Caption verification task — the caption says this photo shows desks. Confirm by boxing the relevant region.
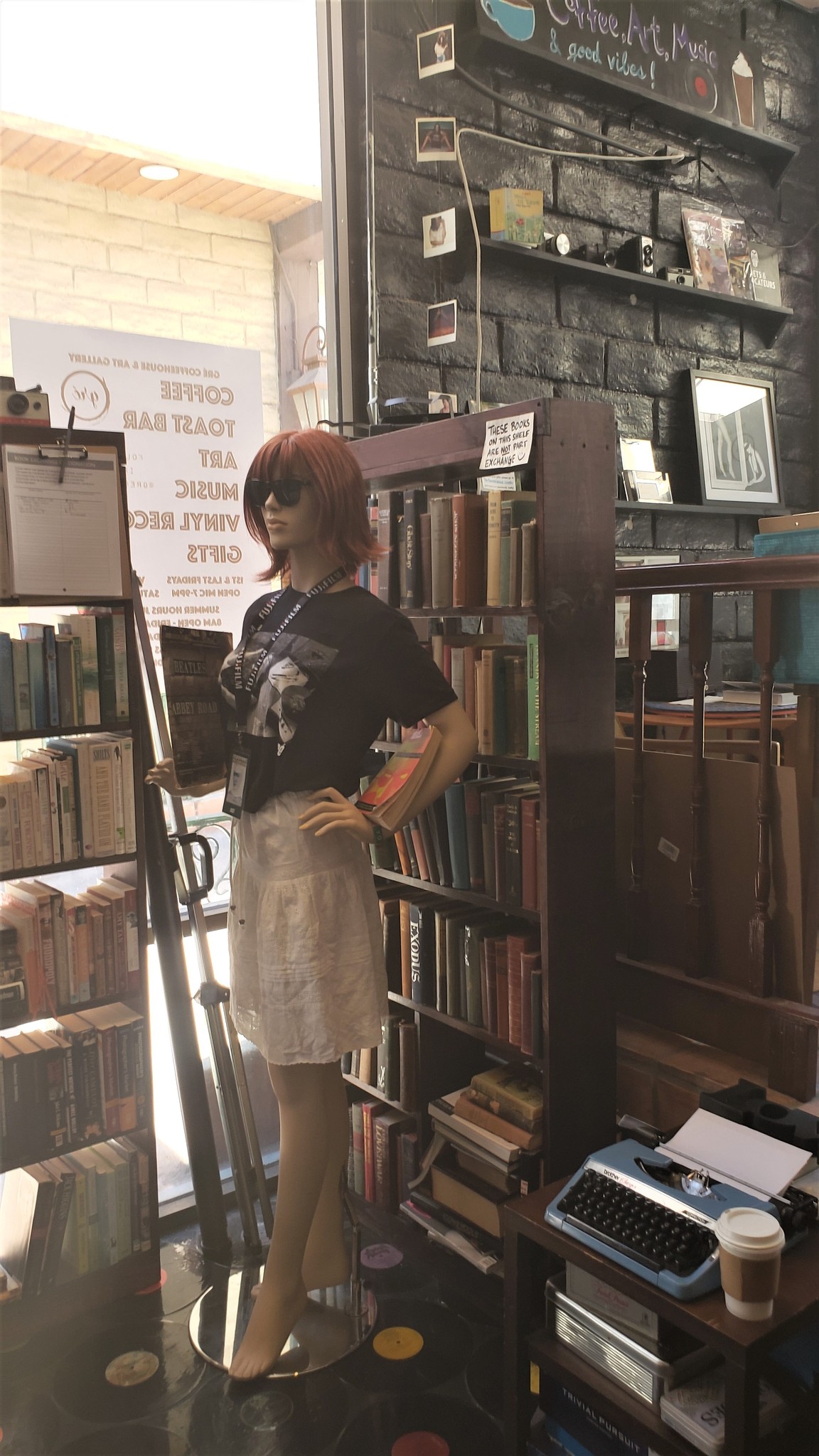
[501,1173,819,1453]
[645,702,800,764]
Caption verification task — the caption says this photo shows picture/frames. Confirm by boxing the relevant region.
[683,369,788,512]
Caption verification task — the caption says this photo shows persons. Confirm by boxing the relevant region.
[419,123,454,152]
[434,31,448,63]
[145,426,478,1383]
[439,399,455,414]
[714,413,769,493]
[429,216,446,248]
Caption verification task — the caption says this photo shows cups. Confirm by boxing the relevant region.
[715,1206,785,1322]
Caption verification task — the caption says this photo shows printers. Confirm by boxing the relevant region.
[545,1115,818,1302]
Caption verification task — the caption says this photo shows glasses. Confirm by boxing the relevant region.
[248,479,315,507]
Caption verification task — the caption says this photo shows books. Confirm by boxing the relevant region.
[376,635,541,762]
[0,611,130,731]
[721,681,800,707]
[0,733,137,874]
[340,1007,417,1114]
[342,1098,418,1206]
[0,1002,146,1160]
[489,187,544,249]
[1,1136,151,1308]
[354,724,444,832]
[355,490,538,609]
[0,878,139,1017]
[400,1060,543,1278]
[348,774,541,912]
[374,883,543,1059]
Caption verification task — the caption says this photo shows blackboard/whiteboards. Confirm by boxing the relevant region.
[476,0,768,136]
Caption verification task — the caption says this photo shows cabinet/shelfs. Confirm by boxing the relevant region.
[3,419,166,1323]
[298,394,618,1288]
[465,25,794,519]
